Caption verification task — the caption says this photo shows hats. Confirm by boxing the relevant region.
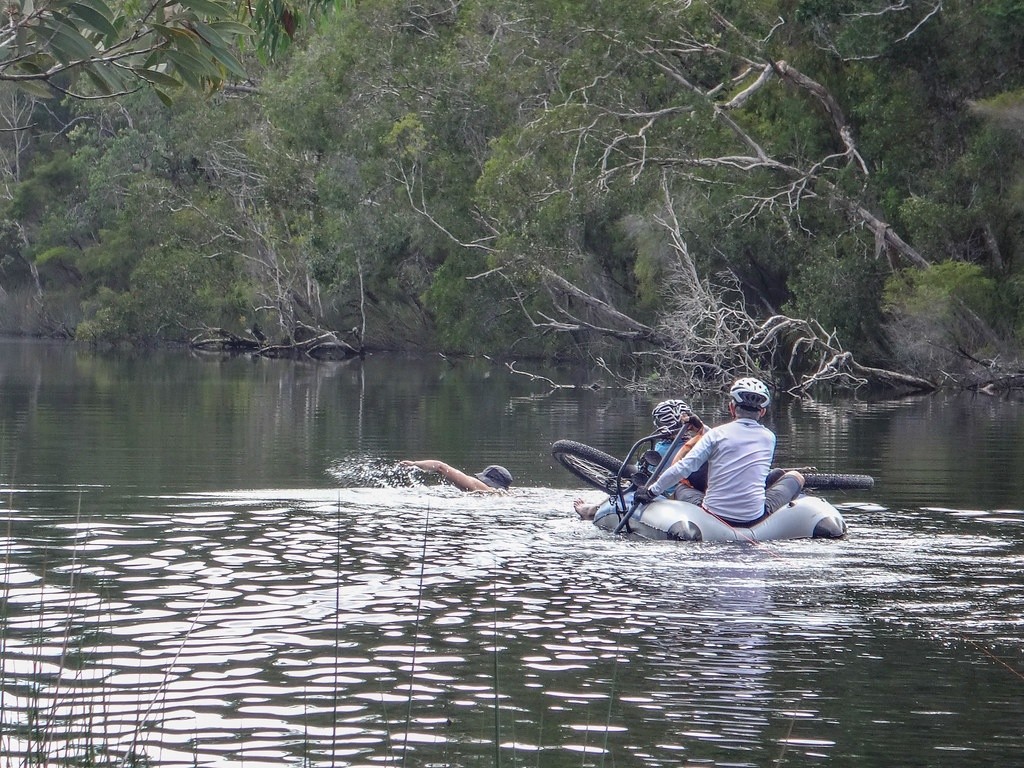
[475,465,513,491]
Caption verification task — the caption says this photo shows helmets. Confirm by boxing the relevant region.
[729,377,770,411]
[652,400,691,434]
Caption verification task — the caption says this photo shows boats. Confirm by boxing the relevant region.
[594,493,850,549]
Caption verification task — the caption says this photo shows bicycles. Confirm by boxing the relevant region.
[550,408,875,536]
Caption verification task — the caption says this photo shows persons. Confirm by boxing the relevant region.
[573,377,805,529]
[399,459,514,493]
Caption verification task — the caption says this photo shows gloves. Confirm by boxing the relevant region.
[634,491,656,505]
